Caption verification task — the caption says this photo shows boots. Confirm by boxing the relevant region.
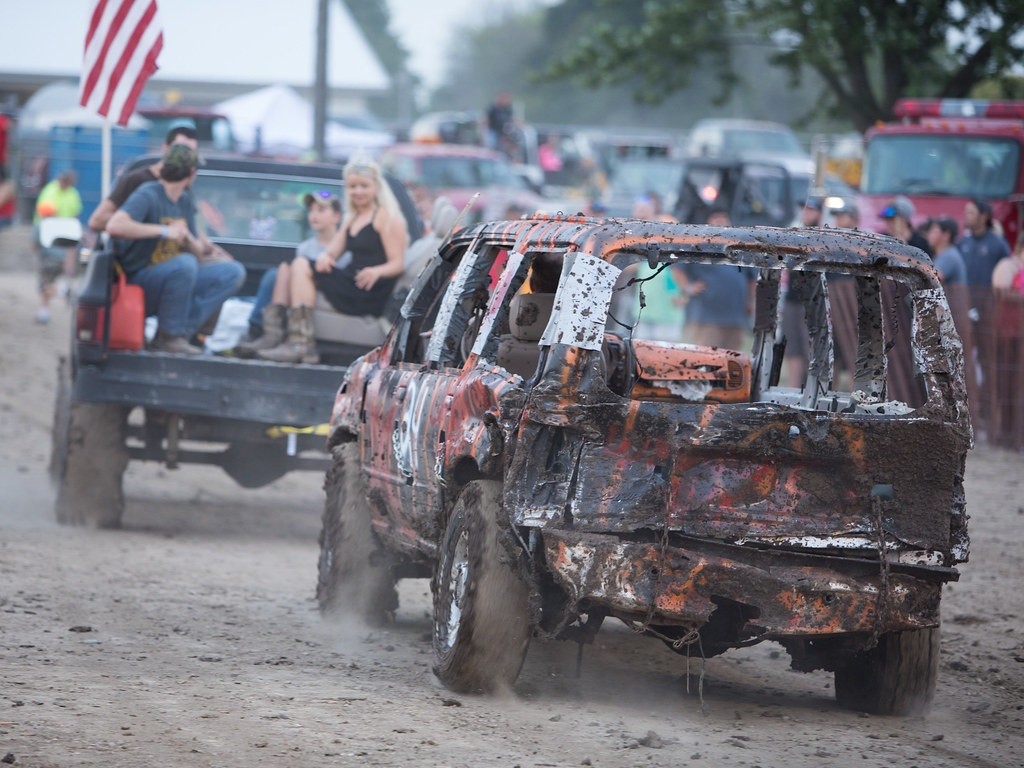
[233,304,287,359]
[256,306,320,364]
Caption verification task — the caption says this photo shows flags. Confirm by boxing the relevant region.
[80,0,164,128]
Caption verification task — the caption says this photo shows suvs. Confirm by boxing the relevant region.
[21,89,854,240]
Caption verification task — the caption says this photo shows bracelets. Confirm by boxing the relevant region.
[161,225,166,239]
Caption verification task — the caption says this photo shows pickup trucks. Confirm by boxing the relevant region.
[50,149,428,528]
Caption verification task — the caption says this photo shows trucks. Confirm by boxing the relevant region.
[317,212,973,717]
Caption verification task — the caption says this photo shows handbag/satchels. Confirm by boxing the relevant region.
[94,264,146,350]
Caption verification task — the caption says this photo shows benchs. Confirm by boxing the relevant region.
[97,284,145,351]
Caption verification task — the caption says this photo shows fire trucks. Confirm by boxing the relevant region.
[856,100,1023,362]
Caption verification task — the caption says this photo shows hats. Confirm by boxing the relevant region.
[304,190,339,208]
[828,200,858,215]
[877,205,911,219]
[798,197,822,209]
[930,213,958,234]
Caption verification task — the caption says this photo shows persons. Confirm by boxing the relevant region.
[32,170,84,322]
[670,191,1024,451]
[106,143,245,355]
[212,192,351,357]
[461,256,563,364]
[482,95,524,164]
[235,158,408,364]
[88,119,223,352]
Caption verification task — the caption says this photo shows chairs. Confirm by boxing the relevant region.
[311,196,458,344]
[493,293,610,384]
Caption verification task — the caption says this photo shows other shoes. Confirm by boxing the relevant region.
[151,334,203,355]
